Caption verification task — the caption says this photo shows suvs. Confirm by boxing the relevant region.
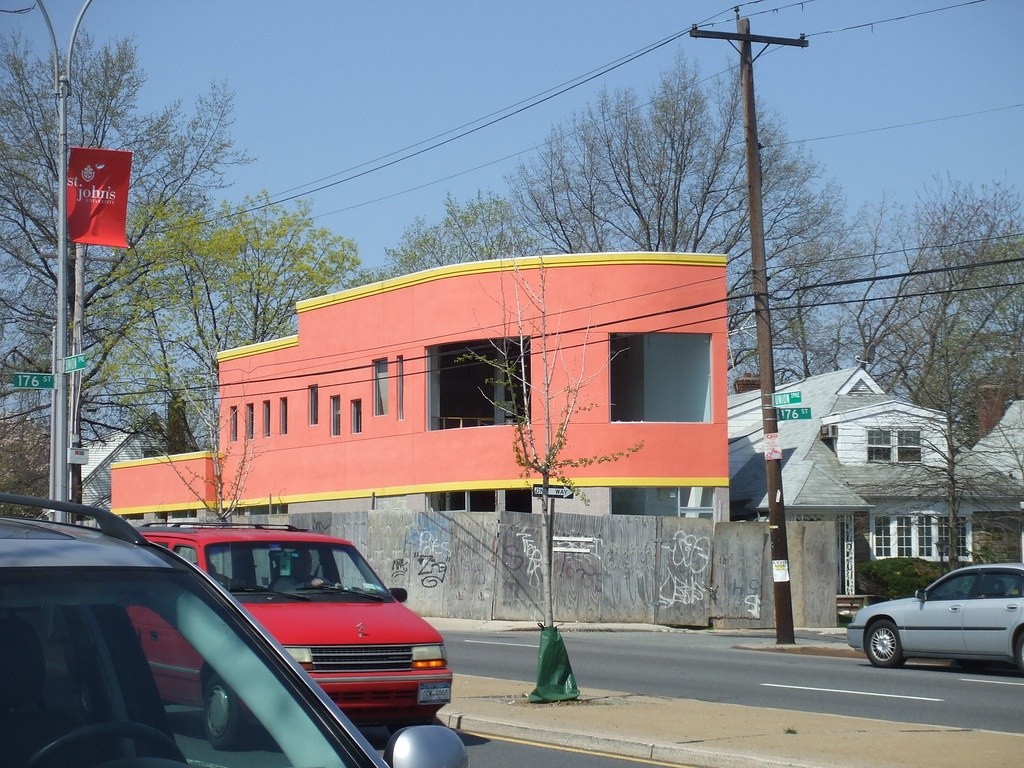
[100,521,454,752]
[1,493,471,767]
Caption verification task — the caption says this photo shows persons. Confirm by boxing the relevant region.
[272,550,342,592]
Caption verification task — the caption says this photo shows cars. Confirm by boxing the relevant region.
[846,562,1024,676]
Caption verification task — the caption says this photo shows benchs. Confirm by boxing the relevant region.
[836,595,875,626]
